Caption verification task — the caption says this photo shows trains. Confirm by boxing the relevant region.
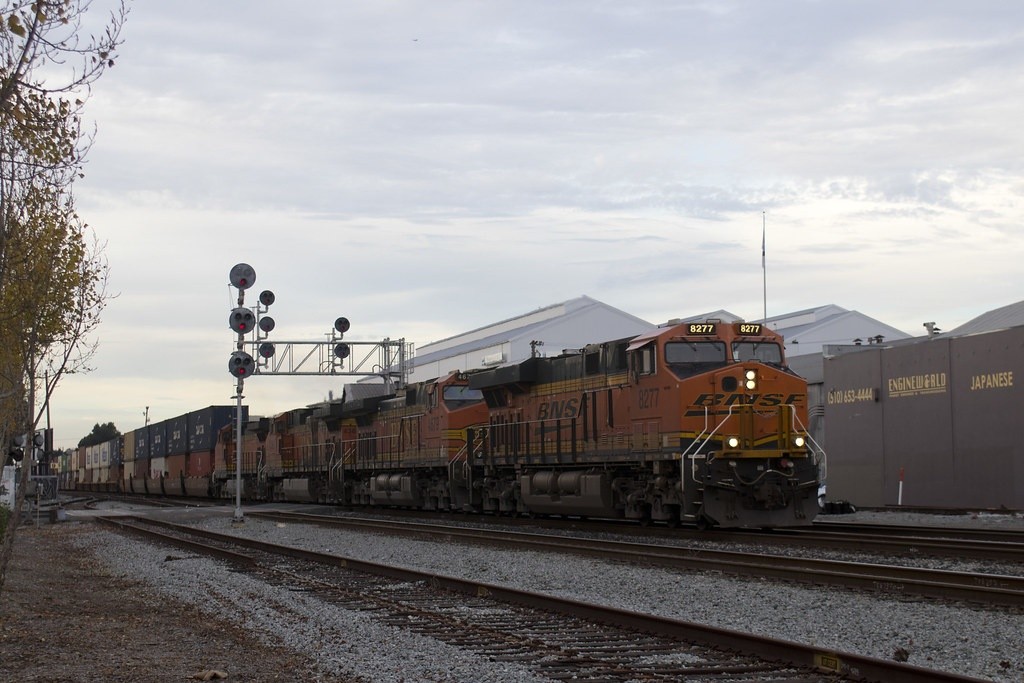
[57,318,826,531]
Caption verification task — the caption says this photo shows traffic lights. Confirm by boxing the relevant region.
[261,317,275,332]
[260,290,275,306]
[335,317,350,332]
[260,343,274,358]
[334,342,349,358]
[228,263,257,290]
[229,309,255,333]
[228,352,256,378]
[7,449,23,462]
